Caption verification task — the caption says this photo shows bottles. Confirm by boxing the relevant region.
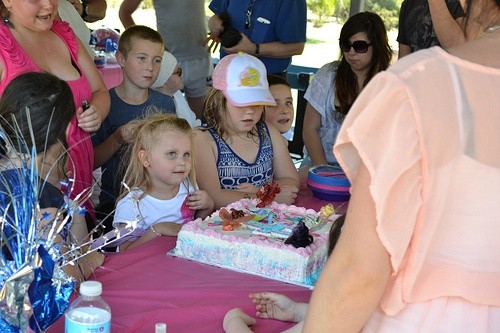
[64,281,111,333]
[106,37,113,52]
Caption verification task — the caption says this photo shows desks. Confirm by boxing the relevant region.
[90,45,123,90]
[26,178,351,333]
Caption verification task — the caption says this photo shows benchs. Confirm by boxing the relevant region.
[208,55,320,159]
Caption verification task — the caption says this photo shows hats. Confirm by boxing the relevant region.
[212,51,277,108]
[150,51,177,89]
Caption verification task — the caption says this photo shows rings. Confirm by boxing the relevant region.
[95,121,99,126]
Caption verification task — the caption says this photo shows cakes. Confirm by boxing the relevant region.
[174,183,344,286]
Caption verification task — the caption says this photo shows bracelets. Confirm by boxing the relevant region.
[149,224,160,238]
[255,44,260,55]
[82,4,89,18]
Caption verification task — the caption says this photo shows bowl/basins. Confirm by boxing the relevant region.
[307,164,351,202]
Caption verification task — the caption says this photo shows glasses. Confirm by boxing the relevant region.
[341,39,373,54]
[172,68,182,77]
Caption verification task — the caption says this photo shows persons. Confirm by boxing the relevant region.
[117,1,214,108]
[223,212,346,332]
[0,72,107,318]
[114,115,213,254]
[300,11,398,168]
[88,27,182,238]
[395,0,466,59]
[206,1,308,83]
[263,74,297,146]
[1,0,114,228]
[300,0,500,332]
[191,51,300,208]
[150,52,203,129]
[57,0,109,58]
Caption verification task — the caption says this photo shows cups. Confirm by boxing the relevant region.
[95,56,106,67]
